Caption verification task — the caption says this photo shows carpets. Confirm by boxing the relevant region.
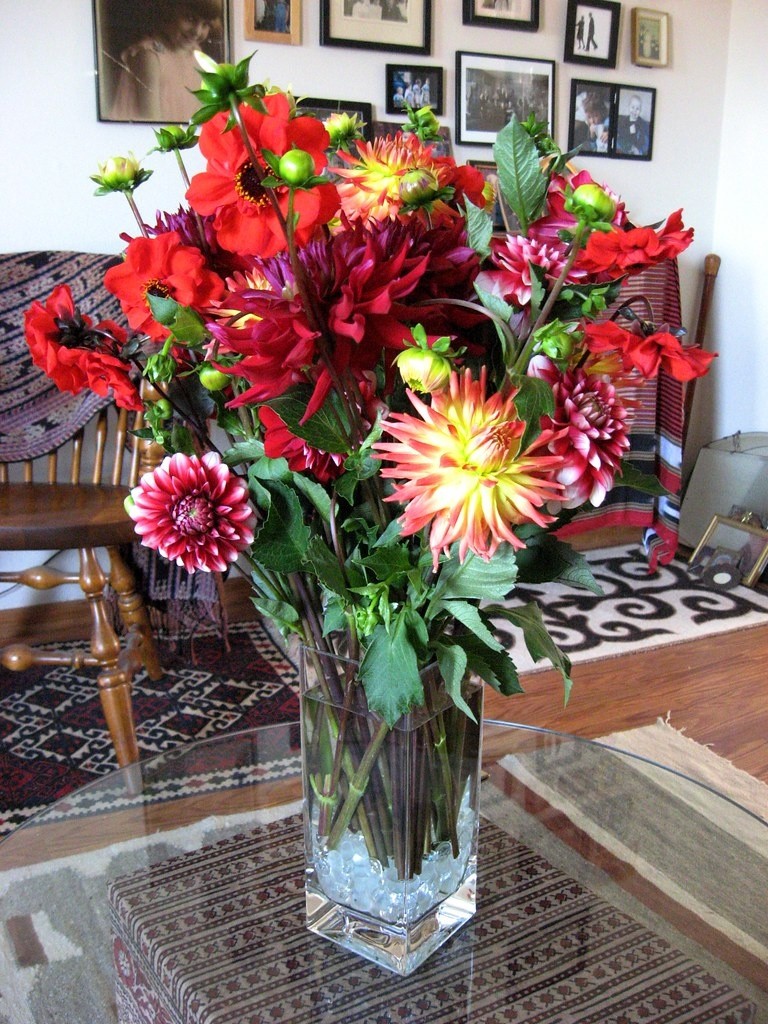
[0,619,302,837]
[0,709,768,1024]
[444,539,768,687]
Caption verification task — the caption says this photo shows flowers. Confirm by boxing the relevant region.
[21,47,722,878]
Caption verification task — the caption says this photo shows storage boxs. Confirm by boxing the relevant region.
[676,431,768,585]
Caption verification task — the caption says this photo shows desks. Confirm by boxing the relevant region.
[0,718,768,1024]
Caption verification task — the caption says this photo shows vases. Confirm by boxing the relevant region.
[297,642,486,976]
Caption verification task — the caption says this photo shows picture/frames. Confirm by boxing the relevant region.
[455,50,556,148]
[466,159,522,231]
[563,0,621,68]
[293,96,373,157]
[611,84,657,161]
[92,0,231,125]
[462,0,539,33]
[319,0,432,55]
[385,63,443,116]
[631,7,671,68]
[243,0,302,46]
[567,78,616,158]
[687,513,768,587]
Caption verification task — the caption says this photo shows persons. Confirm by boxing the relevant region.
[393,77,430,109]
[110,0,223,122]
[574,93,609,153]
[638,25,659,57]
[352,0,401,22]
[615,95,649,155]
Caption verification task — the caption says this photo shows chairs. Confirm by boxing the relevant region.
[0,252,166,801]
